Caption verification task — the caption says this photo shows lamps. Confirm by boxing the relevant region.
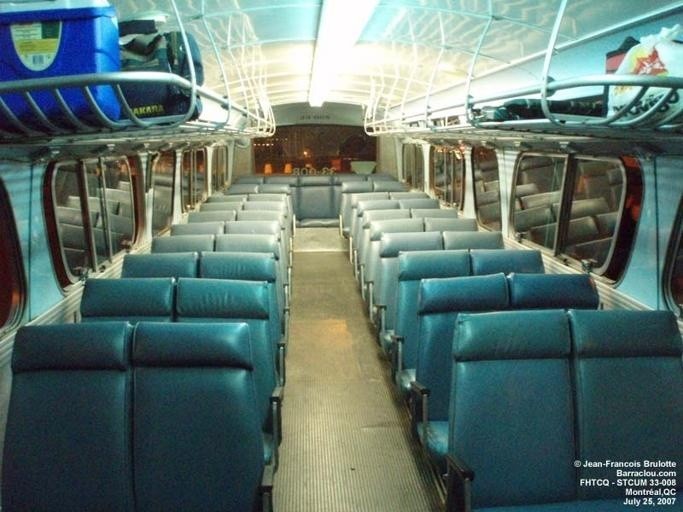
[307,0,381,107]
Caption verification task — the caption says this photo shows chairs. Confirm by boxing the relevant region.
[411,273,508,501]
[79,278,172,324]
[349,192,388,266]
[354,201,399,275]
[443,230,506,252]
[249,194,293,247]
[400,197,439,210]
[568,308,683,512]
[425,218,479,233]
[175,277,277,512]
[243,201,292,248]
[299,175,333,228]
[197,202,243,212]
[392,248,471,411]
[333,173,366,230]
[561,151,624,270]
[149,233,216,253]
[265,175,298,223]
[52,175,139,278]
[169,225,227,240]
[359,210,409,304]
[448,310,628,512]
[234,174,265,184]
[411,208,459,220]
[227,184,258,196]
[339,181,372,240]
[377,232,442,357]
[227,220,288,350]
[391,192,431,201]
[120,253,198,279]
[5,323,133,511]
[214,233,286,391]
[366,173,397,183]
[238,210,292,310]
[507,152,565,252]
[368,218,425,327]
[508,269,615,313]
[469,144,503,230]
[150,156,175,229]
[133,322,265,511]
[187,211,236,224]
[372,181,406,194]
[470,249,545,277]
[259,183,294,231]
[208,196,247,202]
[429,145,464,208]
[199,251,279,454]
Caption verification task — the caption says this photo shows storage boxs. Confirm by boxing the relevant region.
[0,6,122,125]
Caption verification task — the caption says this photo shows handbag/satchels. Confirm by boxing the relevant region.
[117,17,206,125]
